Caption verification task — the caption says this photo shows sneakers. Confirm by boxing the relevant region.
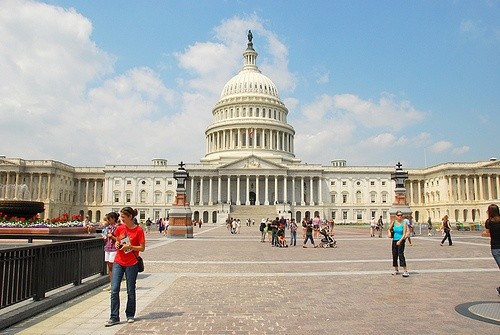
[102,285,112,291]
[104,318,121,326]
[392,270,400,276]
[402,272,410,277]
[495,286,500,297]
[126,315,135,323]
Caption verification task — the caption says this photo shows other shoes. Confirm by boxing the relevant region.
[314,245,318,248]
[303,246,307,248]
[440,242,443,245]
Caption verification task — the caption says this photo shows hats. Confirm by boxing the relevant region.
[260,218,266,223]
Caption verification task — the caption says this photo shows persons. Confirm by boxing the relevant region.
[260,215,335,248]
[388,210,410,276]
[486,204,500,297]
[84,214,91,230]
[370,215,384,238]
[226,217,241,234]
[105,207,145,326]
[156,217,170,233]
[404,215,413,247]
[145,217,153,234]
[248,30,253,42]
[246,218,255,227]
[440,215,454,246]
[427,217,433,237]
[193,218,202,228]
[102,211,121,291]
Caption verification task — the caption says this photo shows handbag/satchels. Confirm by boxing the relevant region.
[136,256,144,272]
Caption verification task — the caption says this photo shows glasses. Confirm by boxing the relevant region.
[396,214,402,216]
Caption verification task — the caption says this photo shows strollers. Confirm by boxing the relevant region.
[317,227,337,248]
[273,227,288,247]
